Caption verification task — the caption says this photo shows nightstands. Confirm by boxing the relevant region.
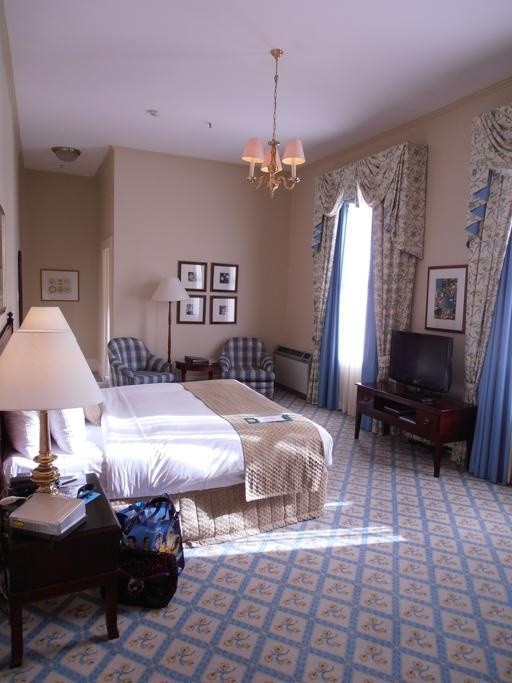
[0,473,123,668]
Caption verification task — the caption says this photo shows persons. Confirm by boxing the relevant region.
[125,534,169,554]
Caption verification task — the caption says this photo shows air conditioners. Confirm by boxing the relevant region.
[274,344,313,396]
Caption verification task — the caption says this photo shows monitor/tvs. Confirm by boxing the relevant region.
[388,329,453,402]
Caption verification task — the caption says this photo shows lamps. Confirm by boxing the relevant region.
[241,48,305,198]
[0,329,103,496]
[52,147,81,162]
[18,306,68,329]
[151,277,190,363]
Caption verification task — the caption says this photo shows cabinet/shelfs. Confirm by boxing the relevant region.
[354,382,476,477]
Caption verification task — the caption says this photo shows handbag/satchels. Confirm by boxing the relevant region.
[100,542,178,609]
[116,493,185,576]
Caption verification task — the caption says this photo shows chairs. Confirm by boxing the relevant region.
[106,337,177,386]
[218,337,275,400]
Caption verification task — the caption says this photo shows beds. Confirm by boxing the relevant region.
[0,312,334,548]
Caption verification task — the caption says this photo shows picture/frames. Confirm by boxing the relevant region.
[178,261,207,292]
[209,295,238,325]
[40,268,80,303]
[424,264,467,333]
[176,295,206,325]
[210,262,239,293]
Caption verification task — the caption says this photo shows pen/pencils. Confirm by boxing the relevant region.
[62,479,77,484]
